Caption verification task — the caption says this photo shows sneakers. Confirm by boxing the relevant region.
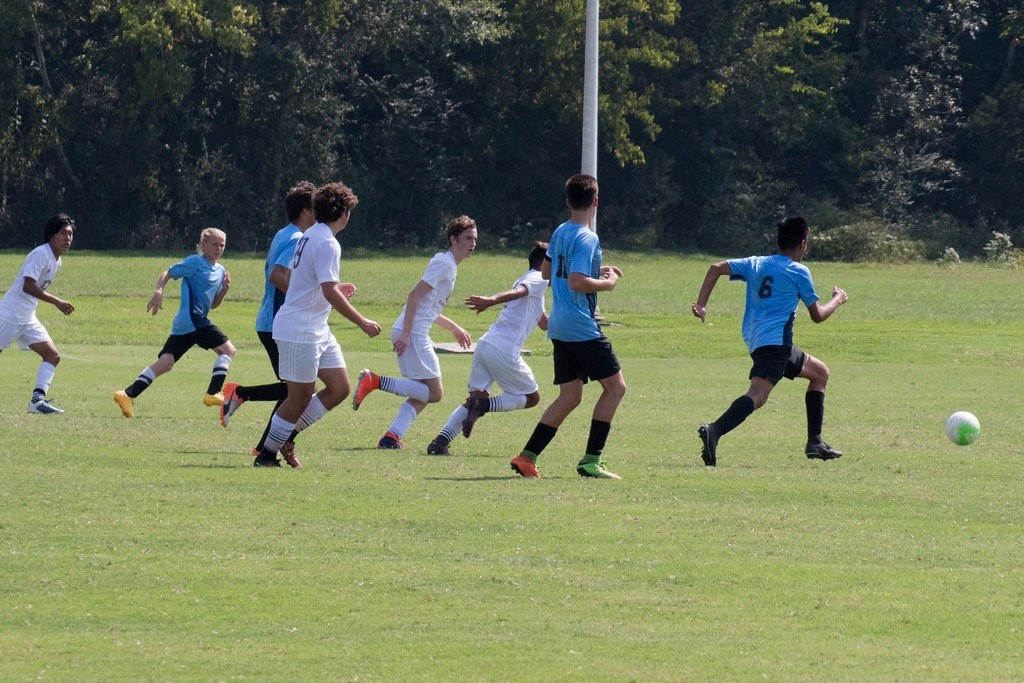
[280,441,303,469]
[426,439,452,457]
[511,453,542,479]
[577,453,623,481]
[376,432,408,450]
[203,392,225,407]
[220,382,245,428]
[27,399,64,416]
[352,368,376,411]
[113,390,135,418]
[252,449,260,457]
[253,454,283,468]
[697,423,719,467]
[804,442,842,461]
[462,395,483,437]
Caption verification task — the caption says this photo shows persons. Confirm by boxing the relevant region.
[113,228,237,417]
[219,181,317,455]
[352,215,478,450]
[691,217,849,466]
[427,240,602,456]
[253,182,383,469]
[510,174,627,482]
[0,213,77,415]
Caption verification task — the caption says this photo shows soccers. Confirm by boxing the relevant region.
[945,410,981,446]
